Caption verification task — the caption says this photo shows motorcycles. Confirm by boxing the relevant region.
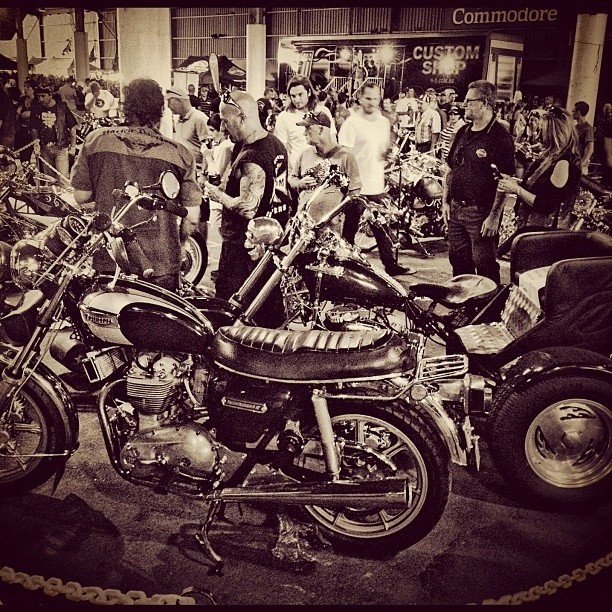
[354,148,528,252]
[0,148,208,286]
[0,167,479,577]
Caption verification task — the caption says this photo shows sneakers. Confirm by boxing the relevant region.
[389,265,417,276]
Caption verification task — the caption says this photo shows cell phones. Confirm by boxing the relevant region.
[491,164,503,179]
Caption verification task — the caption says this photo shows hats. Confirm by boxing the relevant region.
[164,86,190,100]
[295,110,331,128]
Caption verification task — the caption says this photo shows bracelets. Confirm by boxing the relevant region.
[516,185,522,197]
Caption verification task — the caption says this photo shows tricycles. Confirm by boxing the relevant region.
[181,168,610,508]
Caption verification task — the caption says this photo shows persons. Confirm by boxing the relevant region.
[255,88,289,134]
[202,90,290,333]
[1,70,19,146]
[272,75,336,188]
[313,75,358,125]
[496,95,560,157]
[68,78,199,297]
[200,115,231,301]
[599,102,611,189]
[337,85,410,276]
[442,79,516,295]
[349,56,379,91]
[286,111,362,237]
[498,110,578,252]
[59,75,79,156]
[186,84,198,110]
[18,80,37,166]
[199,88,211,111]
[570,100,595,175]
[24,66,91,92]
[382,83,463,167]
[83,82,119,121]
[27,88,75,185]
[166,84,212,292]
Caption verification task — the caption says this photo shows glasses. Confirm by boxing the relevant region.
[549,106,565,118]
[223,89,245,117]
[464,98,483,103]
[166,90,181,96]
[303,113,323,125]
